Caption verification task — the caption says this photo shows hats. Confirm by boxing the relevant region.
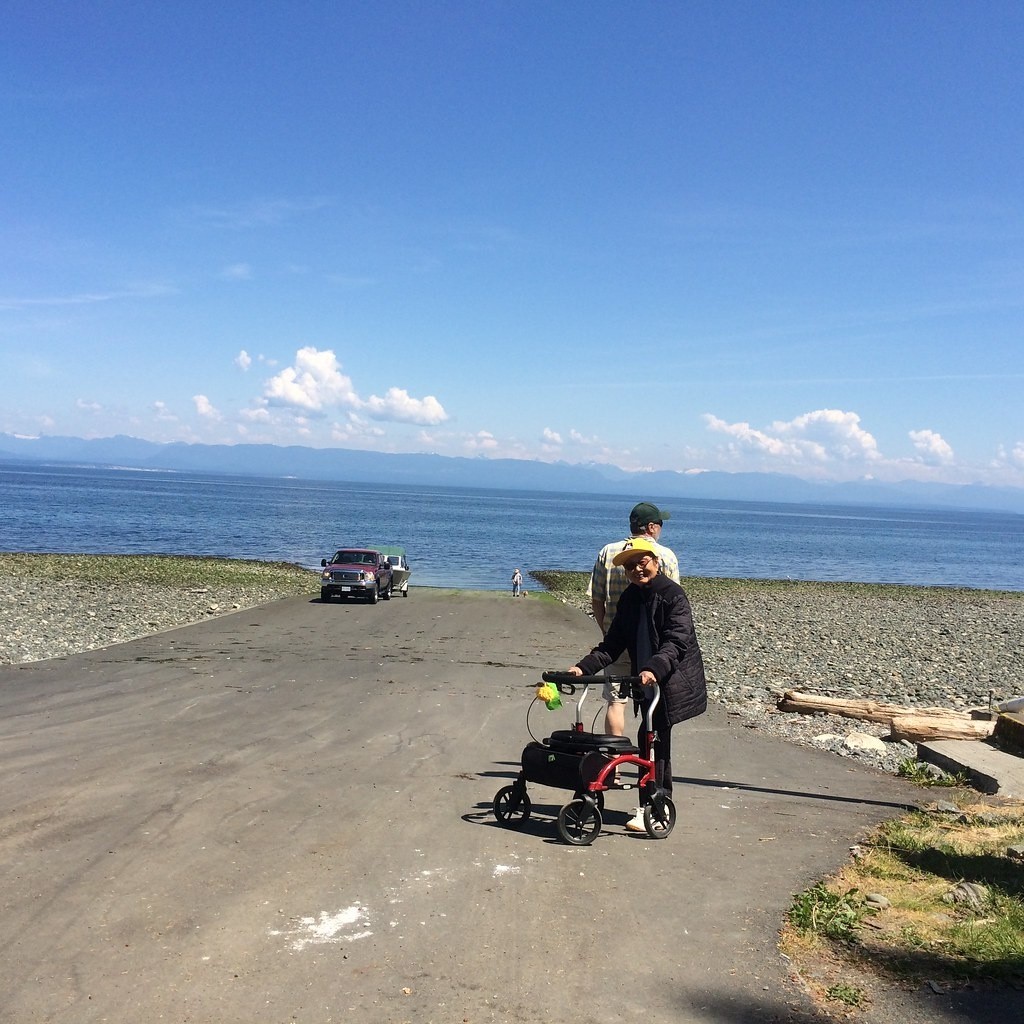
[630,503,670,526]
[514,569,519,574]
[612,538,660,566]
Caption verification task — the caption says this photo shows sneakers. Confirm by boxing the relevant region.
[657,795,672,830]
[627,807,656,831]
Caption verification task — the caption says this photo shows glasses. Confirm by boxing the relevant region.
[624,557,654,572]
[645,519,663,529]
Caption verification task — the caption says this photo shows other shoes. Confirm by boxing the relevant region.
[517,595,519,597]
[513,595,515,596]
[613,772,621,786]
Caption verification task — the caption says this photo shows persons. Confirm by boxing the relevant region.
[567,538,708,832]
[343,555,352,562]
[367,554,375,563]
[511,569,522,596]
[592,502,680,783]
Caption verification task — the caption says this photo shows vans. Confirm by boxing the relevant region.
[367,546,409,597]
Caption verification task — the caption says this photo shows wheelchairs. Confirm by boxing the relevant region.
[493,671,676,845]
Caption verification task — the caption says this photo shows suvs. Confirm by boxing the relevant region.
[322,549,393,602]
